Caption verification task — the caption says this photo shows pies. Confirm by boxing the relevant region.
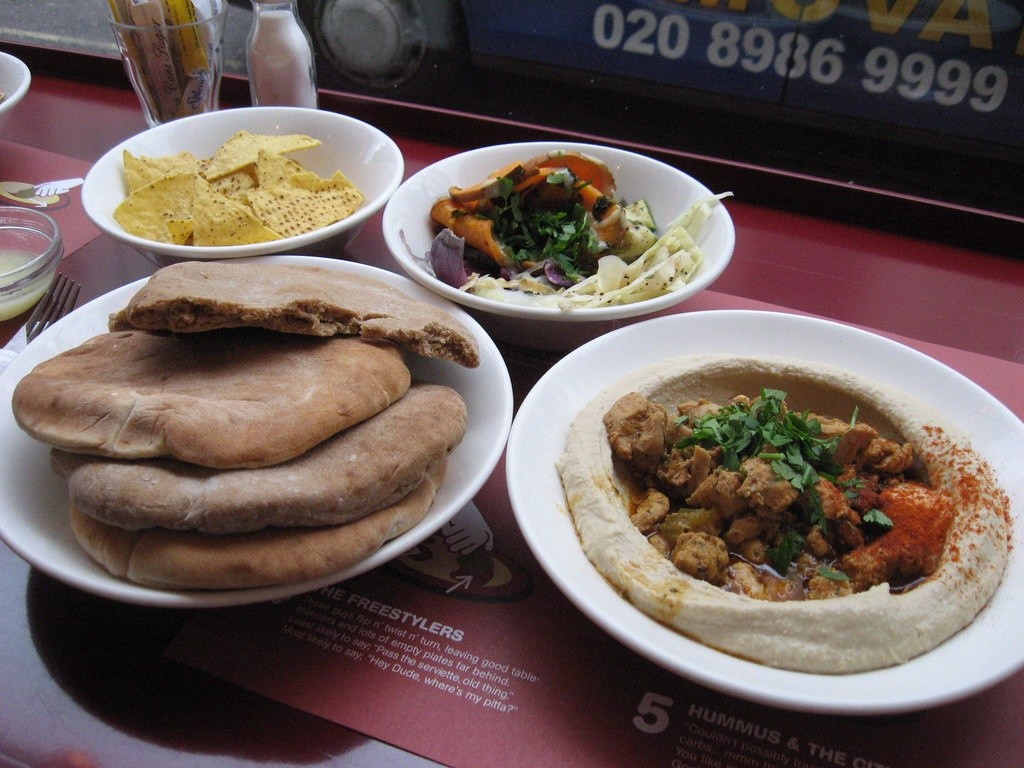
[10,259,480,590]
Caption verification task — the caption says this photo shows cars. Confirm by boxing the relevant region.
[226,1,1024,221]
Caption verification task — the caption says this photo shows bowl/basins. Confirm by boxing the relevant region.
[0,254,514,610]
[382,142,737,323]
[0,51,32,115]
[81,106,404,272]
[0,206,64,322]
[506,309,1023,717]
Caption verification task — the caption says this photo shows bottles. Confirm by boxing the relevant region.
[244,0,319,110]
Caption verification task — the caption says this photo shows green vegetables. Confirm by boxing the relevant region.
[674,385,894,581]
[450,152,598,284]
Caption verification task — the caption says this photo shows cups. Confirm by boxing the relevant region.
[102,0,228,128]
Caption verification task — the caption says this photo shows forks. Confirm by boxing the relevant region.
[0,271,83,373]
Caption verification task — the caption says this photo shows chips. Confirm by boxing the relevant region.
[115,130,364,246]
